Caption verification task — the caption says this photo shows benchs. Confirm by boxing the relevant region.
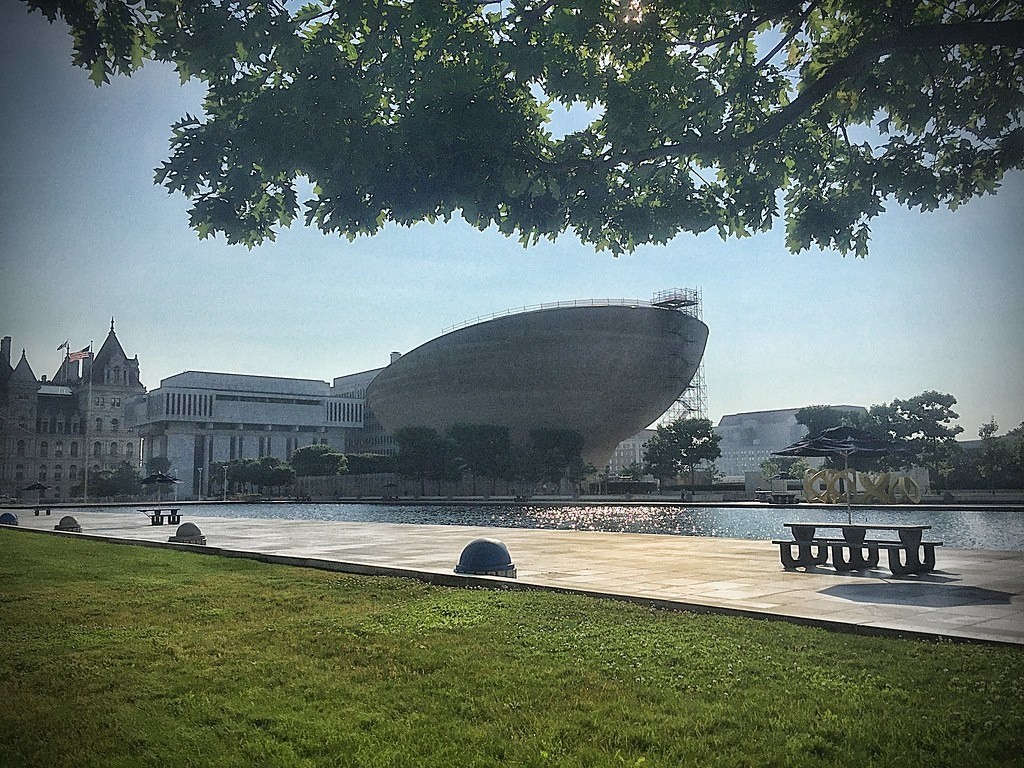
[149,514,183,525]
[768,498,799,504]
[771,536,944,574]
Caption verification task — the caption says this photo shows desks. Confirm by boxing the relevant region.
[137,508,180,525]
[771,495,796,504]
[783,523,932,574]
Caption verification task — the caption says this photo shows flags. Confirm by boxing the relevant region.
[57,340,70,351]
[69,345,90,363]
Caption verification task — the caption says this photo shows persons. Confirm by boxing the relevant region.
[238,487,411,501]
[540,481,763,502]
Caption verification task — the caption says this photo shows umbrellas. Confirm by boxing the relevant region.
[769,472,800,492]
[516,478,533,494]
[769,423,913,523]
[218,487,229,496]
[21,481,53,510]
[382,482,399,497]
[134,470,186,509]
